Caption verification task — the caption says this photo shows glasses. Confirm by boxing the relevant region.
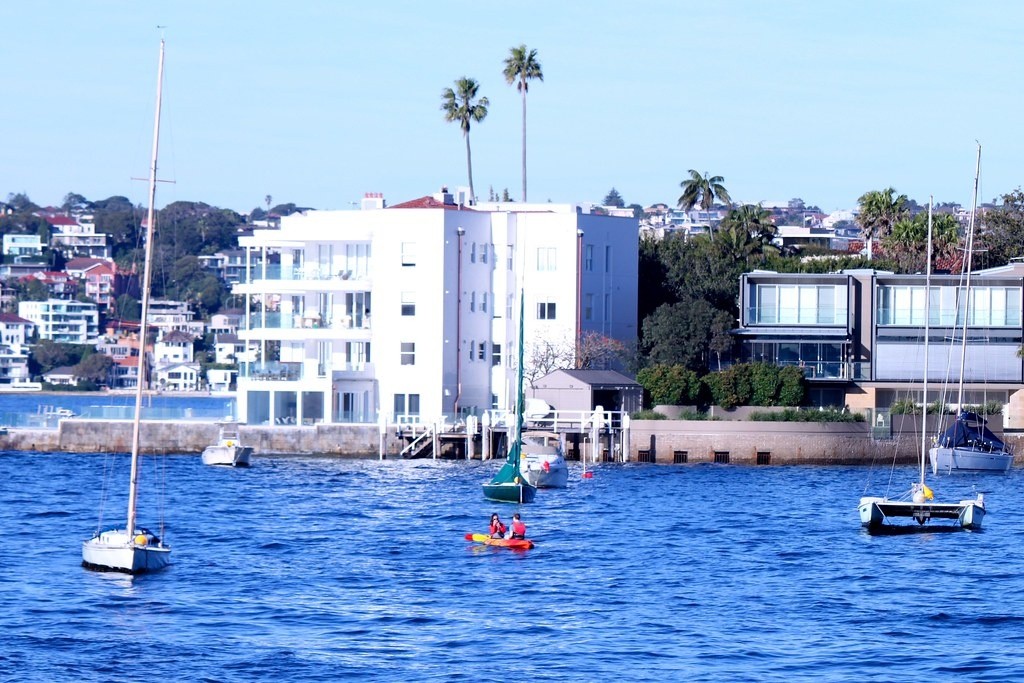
[493,518,498,520]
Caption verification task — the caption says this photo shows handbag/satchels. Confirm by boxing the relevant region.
[498,532,504,538]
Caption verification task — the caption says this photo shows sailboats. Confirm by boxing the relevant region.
[482,278,537,504]
[83,25,172,574]
[856,194,988,531]
[928,139,1015,473]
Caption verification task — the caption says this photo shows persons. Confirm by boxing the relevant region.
[490,513,506,539]
[505,514,526,540]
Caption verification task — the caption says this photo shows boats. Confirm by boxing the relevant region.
[465,531,533,551]
[516,430,570,487]
[200,422,257,468]
